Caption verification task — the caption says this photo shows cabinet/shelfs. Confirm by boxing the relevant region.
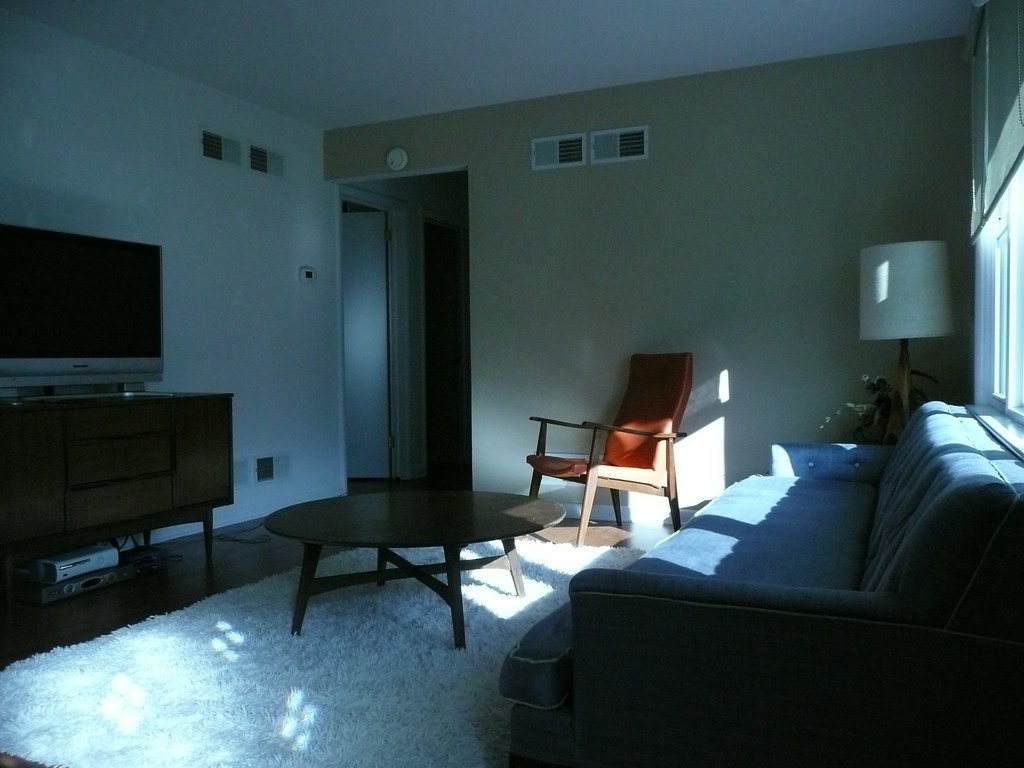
[0,390,234,626]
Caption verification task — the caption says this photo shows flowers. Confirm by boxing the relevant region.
[820,367,949,443]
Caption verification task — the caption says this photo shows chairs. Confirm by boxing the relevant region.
[529,351,695,548]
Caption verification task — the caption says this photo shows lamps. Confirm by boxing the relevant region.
[860,240,954,435]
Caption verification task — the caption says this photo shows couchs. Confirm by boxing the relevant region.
[500,400,1024,768]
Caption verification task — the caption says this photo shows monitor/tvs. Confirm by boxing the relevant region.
[0,221,174,405]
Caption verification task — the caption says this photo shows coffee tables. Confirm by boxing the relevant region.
[264,492,566,651]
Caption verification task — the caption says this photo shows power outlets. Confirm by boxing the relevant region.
[258,456,275,482]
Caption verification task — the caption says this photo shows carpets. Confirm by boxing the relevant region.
[0,534,646,768]
